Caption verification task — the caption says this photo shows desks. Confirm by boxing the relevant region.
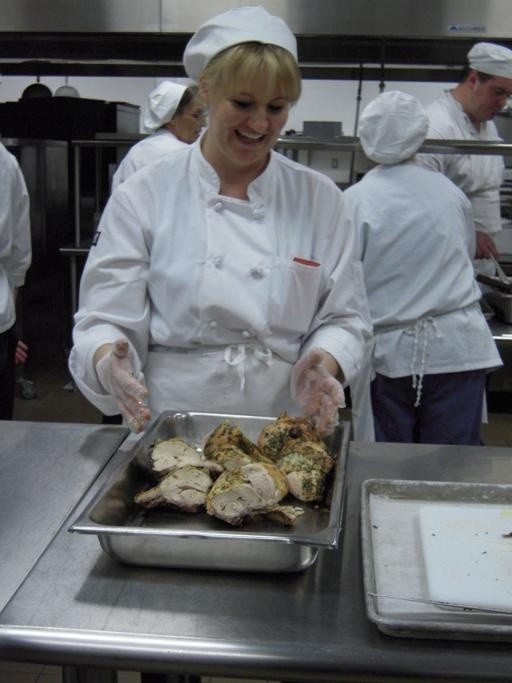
[2,425,506,678]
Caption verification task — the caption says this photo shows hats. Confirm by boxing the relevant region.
[180,6,298,86]
[467,42,512,79]
[358,90,430,164]
[139,80,190,135]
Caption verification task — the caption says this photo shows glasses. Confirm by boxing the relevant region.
[177,111,209,119]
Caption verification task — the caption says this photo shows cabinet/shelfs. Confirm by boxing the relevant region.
[56,130,512,393]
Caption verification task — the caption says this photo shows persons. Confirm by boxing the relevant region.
[413,42,512,322]
[69,6,374,437]
[111,80,206,198]
[342,88,503,443]
[0,137,32,418]
